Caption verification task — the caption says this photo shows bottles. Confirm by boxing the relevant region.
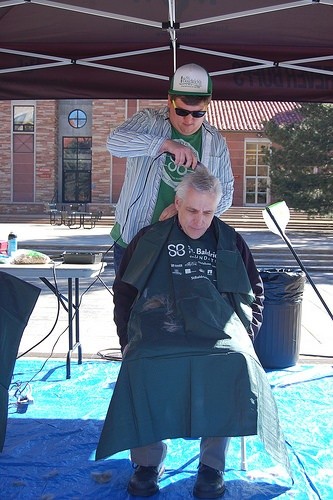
[8,232,17,256]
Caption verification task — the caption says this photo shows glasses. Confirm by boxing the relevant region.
[170,94,209,118]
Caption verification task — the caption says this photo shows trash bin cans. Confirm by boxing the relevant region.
[253,266,307,368]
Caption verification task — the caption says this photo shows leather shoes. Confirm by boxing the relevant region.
[127,461,164,495]
[192,463,226,499]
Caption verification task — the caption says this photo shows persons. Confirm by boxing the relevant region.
[105,64,235,358]
[111,170,268,499]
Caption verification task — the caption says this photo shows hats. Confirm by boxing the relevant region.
[168,62,212,96]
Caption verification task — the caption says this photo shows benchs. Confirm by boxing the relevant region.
[47,204,103,229]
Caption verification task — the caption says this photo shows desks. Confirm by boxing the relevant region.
[73,212,92,228]
[0,260,107,380]
